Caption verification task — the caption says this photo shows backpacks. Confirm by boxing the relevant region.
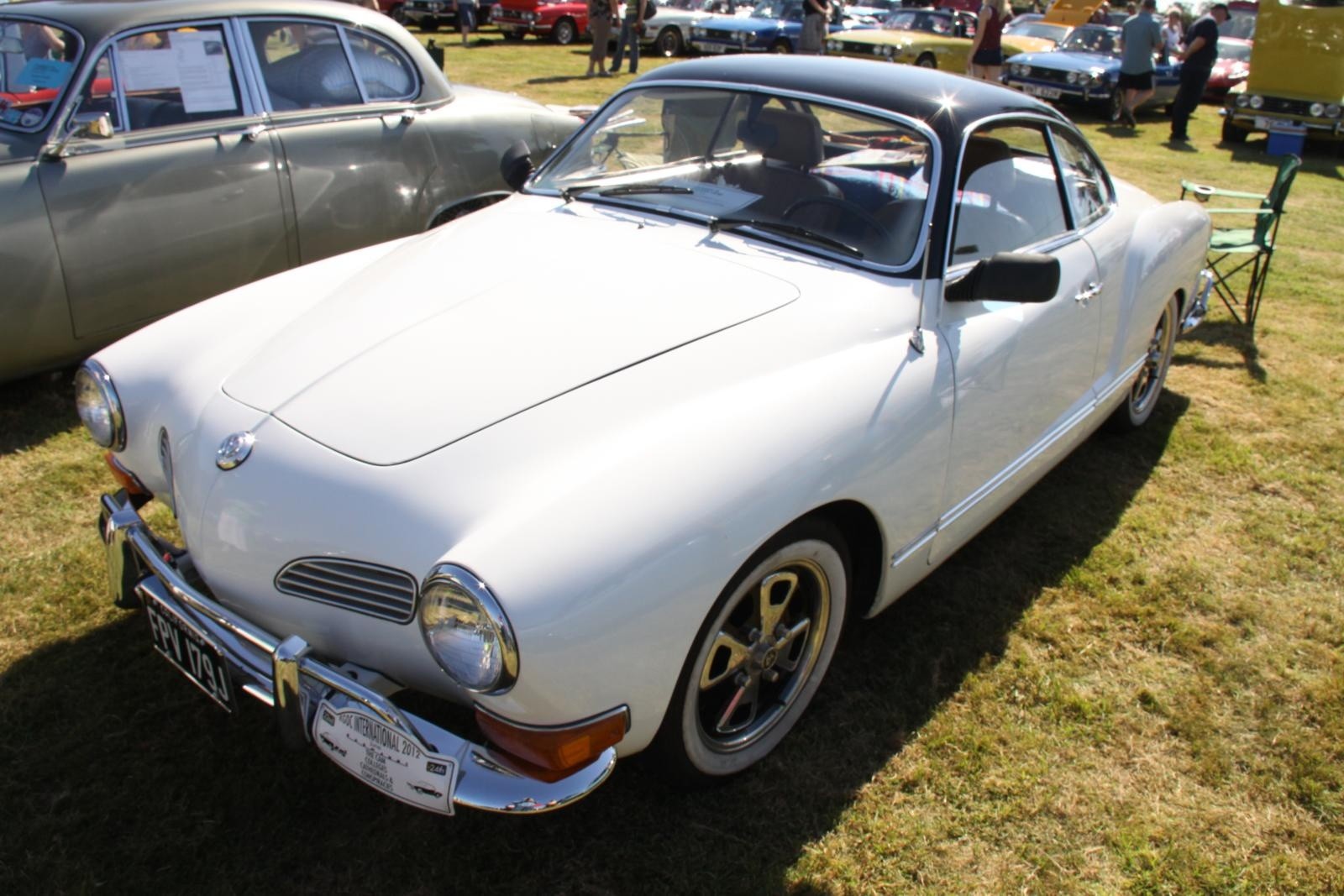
[638,0,657,20]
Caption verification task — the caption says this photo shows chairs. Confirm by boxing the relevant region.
[864,135,1034,269]
[1181,149,1302,330]
[710,108,845,233]
[1097,39,1116,54]
[123,96,243,129]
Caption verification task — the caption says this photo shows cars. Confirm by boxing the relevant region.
[686,0,890,58]
[371,0,495,34]
[486,0,595,45]
[1222,0,1344,146]
[998,0,1130,58]
[0,0,592,391]
[73,49,1212,826]
[1203,0,1259,102]
[585,0,717,59]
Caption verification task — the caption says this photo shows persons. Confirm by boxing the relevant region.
[1092,1,1112,26]
[587,0,621,77]
[289,0,380,49]
[1126,2,1136,15]
[1119,0,1163,129]
[799,0,833,55]
[968,0,1013,81]
[1161,7,1180,53]
[453,0,480,45]
[1169,3,1231,140]
[608,0,647,73]
[21,23,65,60]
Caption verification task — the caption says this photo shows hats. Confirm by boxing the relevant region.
[1211,4,1231,22]
[1142,0,1156,8]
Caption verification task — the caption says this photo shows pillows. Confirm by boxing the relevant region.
[262,43,411,106]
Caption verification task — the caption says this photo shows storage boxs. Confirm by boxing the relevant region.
[1267,120,1308,157]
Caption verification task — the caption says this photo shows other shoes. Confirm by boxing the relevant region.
[587,73,598,78]
[1170,134,1189,142]
[598,72,612,77]
[629,70,637,74]
[1119,107,1136,128]
[609,68,618,73]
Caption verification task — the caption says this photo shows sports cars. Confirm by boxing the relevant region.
[822,6,981,74]
[996,23,1190,113]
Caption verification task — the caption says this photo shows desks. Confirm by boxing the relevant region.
[598,114,664,172]
